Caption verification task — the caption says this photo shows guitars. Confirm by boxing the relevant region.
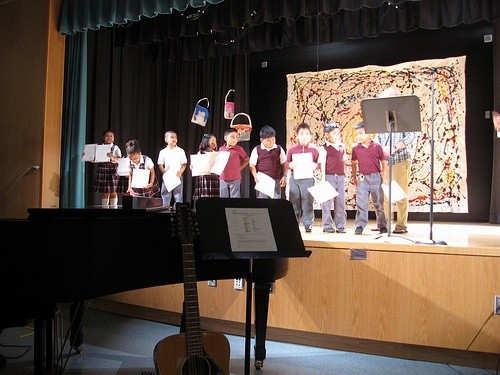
[153,202,232,375]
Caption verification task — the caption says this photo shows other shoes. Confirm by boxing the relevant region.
[380,227,387,233]
[323,227,335,233]
[305,225,312,232]
[355,226,363,235]
[336,227,347,233]
[371,228,379,231]
[393,227,406,233]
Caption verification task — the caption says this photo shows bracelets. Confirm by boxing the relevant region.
[393,145,398,151]
[283,176,286,179]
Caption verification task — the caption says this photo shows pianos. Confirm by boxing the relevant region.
[0,197,312,375]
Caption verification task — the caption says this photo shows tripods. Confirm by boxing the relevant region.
[360,95,421,239]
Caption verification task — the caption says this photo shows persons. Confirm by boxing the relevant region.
[351,121,388,235]
[157,131,187,207]
[371,89,415,232]
[249,126,287,199]
[190,135,220,200]
[219,128,249,198]
[286,124,319,232]
[317,123,351,233]
[126,140,160,198]
[82,130,122,208]
